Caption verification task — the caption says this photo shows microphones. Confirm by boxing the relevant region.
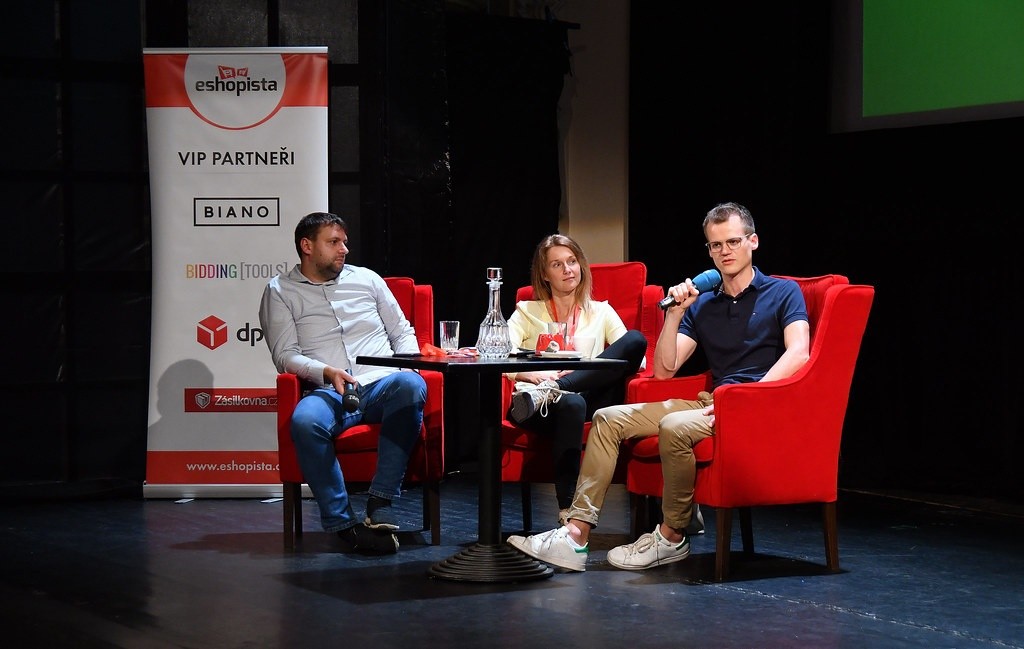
[342,369,360,414]
[657,269,721,310]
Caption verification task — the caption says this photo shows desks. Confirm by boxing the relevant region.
[355,353,629,584]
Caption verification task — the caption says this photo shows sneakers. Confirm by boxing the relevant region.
[506,526,589,571]
[362,496,400,530]
[558,508,569,527]
[607,524,691,570]
[337,522,400,557]
[510,379,582,423]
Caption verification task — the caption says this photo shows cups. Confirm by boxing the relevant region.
[548,322,567,339]
[440,321,460,351]
[574,336,596,358]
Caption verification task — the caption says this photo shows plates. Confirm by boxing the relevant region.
[540,350,582,357]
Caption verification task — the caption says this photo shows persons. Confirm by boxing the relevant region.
[258,212,428,554]
[503,234,647,524]
[507,203,810,572]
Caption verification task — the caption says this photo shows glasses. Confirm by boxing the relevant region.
[705,232,754,253]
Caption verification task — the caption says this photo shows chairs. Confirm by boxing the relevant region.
[502,260,665,530]
[626,274,876,581]
[277,277,445,553]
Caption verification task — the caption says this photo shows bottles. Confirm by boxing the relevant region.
[475,267,513,360]
